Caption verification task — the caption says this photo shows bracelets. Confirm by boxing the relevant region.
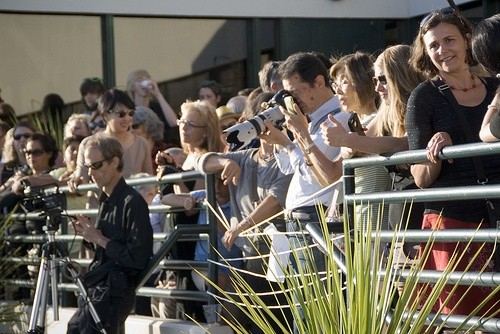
[305,141,315,154]
[306,161,313,166]
[284,141,294,151]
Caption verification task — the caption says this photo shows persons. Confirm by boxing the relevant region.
[1,9,500,332]
[256,52,355,334]
[195,92,298,334]
[65,136,154,334]
[406,9,499,332]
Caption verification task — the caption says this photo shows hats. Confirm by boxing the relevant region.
[126,70,150,103]
[215,106,242,120]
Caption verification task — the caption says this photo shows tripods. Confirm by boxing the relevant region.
[27,211,107,334]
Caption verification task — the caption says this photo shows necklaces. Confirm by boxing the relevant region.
[438,74,476,92]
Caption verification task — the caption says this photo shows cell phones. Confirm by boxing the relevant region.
[348,113,366,136]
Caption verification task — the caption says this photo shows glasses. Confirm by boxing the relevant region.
[89,119,106,131]
[106,109,134,118]
[13,132,33,140]
[176,118,208,129]
[83,77,101,83]
[22,147,46,157]
[330,79,351,93]
[266,61,279,89]
[372,74,387,86]
[419,7,464,48]
[82,157,107,170]
[131,118,146,129]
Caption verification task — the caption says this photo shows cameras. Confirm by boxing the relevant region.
[222,87,305,152]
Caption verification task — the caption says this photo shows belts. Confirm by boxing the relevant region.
[283,205,328,220]
